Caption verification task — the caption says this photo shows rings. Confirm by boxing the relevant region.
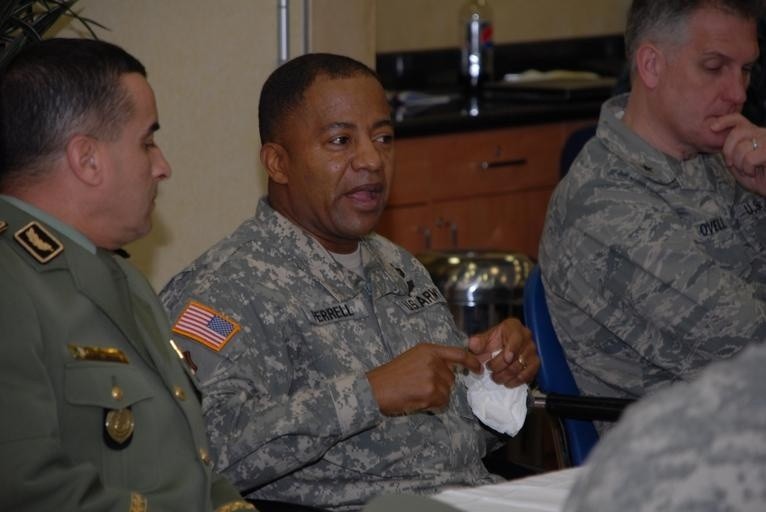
[751,137,757,150]
[516,355,528,368]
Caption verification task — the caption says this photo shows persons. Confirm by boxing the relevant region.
[537,0,766,440]
[0,36,257,511]
[559,343,766,511]
[157,51,541,512]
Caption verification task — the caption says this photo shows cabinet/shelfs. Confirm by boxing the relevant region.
[377,77,599,260]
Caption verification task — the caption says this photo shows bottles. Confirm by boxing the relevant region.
[458,0,495,90]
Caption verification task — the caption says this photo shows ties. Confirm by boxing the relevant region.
[96,246,134,319]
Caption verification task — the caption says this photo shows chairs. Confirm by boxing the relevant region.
[523,267,636,468]
